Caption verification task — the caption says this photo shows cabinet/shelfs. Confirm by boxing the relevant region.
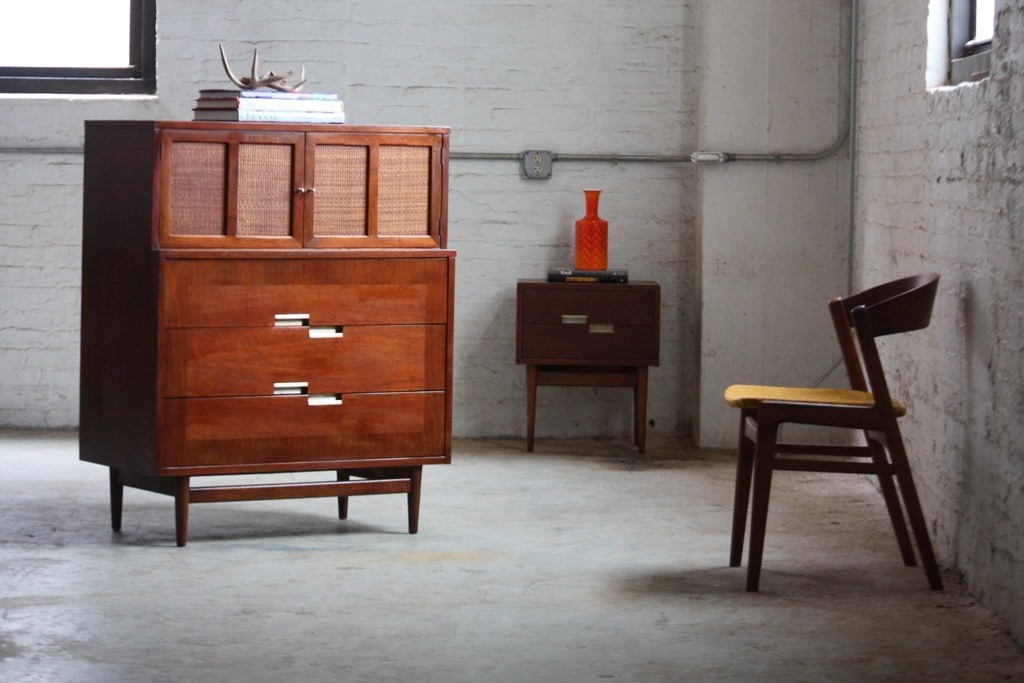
[514,280,662,455]
[78,118,458,549]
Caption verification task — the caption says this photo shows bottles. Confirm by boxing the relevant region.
[574,188,608,271]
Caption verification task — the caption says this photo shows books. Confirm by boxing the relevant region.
[192,89,346,123]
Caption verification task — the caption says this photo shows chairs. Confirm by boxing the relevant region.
[724,272,952,594]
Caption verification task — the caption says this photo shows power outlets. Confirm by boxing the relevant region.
[522,149,552,179]
